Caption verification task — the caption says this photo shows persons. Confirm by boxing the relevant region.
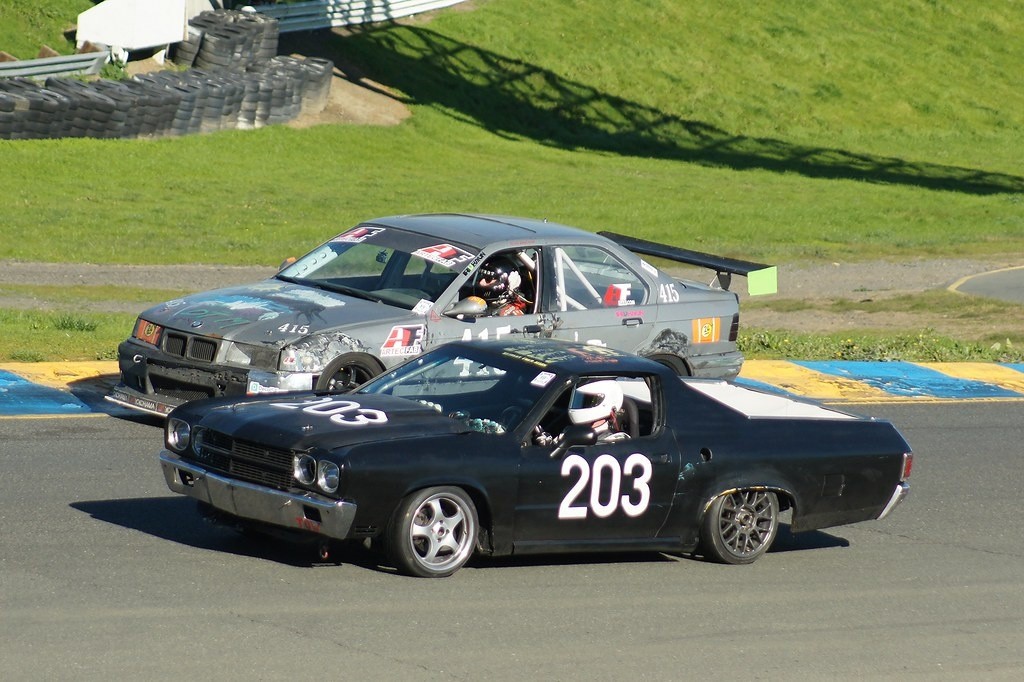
[536,378,631,446]
[472,256,527,317]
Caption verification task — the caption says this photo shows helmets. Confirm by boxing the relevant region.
[476,256,521,304]
[567,379,623,423]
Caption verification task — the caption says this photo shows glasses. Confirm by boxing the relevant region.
[479,273,498,283]
[582,398,598,404]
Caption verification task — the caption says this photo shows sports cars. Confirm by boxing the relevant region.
[103,212,781,421]
[154,335,916,579]
